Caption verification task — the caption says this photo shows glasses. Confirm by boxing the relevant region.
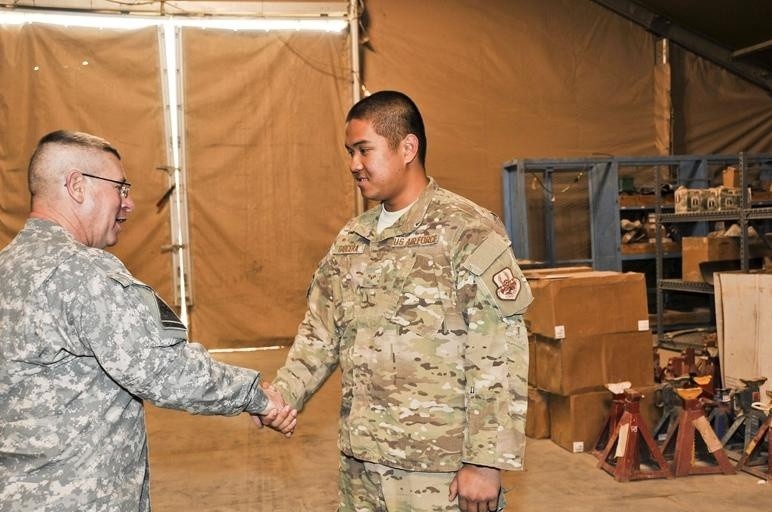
[63,172,131,199]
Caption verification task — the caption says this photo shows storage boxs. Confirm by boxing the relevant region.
[518,267,648,340]
[519,330,661,455]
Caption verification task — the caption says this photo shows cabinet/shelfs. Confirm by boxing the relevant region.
[655,155,772,349]
[503,159,738,326]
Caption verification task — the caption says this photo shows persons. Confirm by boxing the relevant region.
[0,129,296,511]
[250,91,535,511]
